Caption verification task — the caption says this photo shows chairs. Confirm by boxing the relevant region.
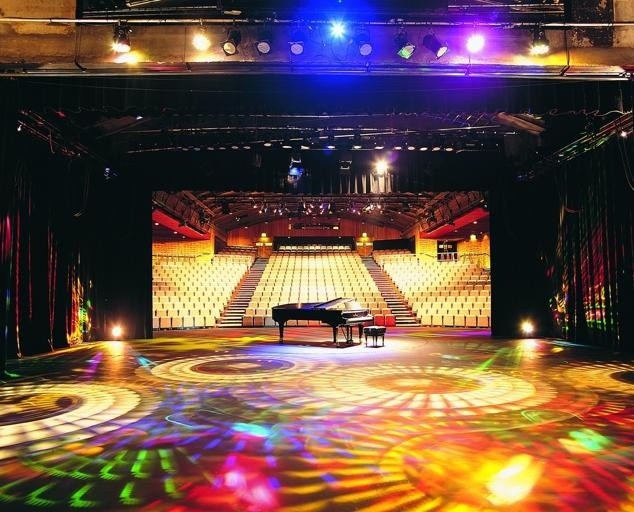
[152,242,493,331]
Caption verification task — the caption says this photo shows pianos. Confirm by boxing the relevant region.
[272,297,374,347]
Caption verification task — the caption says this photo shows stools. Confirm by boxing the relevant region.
[363,326,387,347]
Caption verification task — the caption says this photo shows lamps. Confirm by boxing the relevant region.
[460,27,487,56]
[388,24,417,59]
[527,23,550,58]
[194,26,212,52]
[351,27,375,59]
[421,26,448,61]
[110,24,134,54]
[287,27,308,59]
[219,29,242,57]
[252,27,275,56]
[177,130,456,153]
